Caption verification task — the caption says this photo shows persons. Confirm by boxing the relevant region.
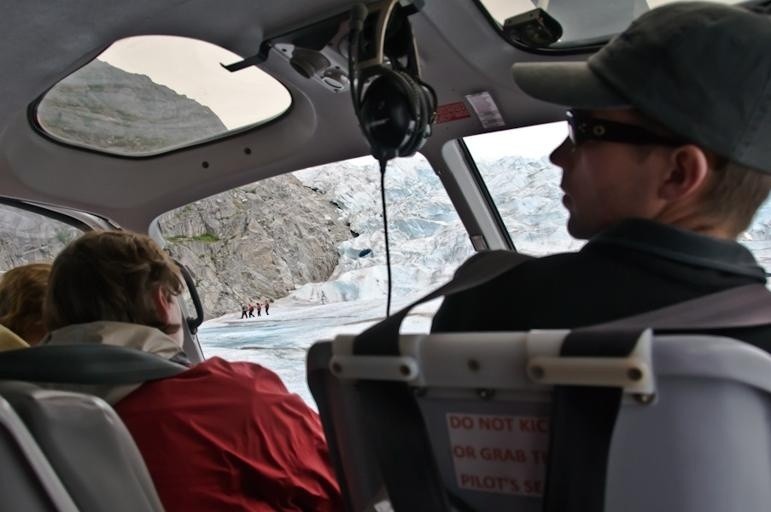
[429,0,770,356]
[247,303,256,318]
[255,302,262,317]
[0,262,54,353]
[30,226,345,512]
[239,301,249,319]
[264,300,270,316]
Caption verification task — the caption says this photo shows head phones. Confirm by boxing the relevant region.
[348,1,438,162]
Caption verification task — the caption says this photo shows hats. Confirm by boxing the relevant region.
[512,0,771,175]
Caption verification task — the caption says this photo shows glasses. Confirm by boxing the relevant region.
[563,108,694,146]
